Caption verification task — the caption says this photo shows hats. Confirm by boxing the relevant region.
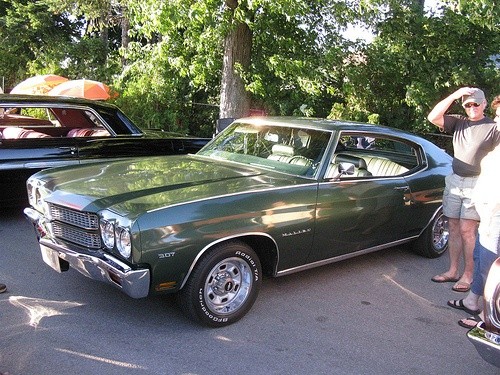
[462,88,485,105]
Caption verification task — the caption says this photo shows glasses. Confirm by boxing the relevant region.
[464,103,480,108]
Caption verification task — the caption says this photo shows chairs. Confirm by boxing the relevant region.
[266,144,411,177]
[3,126,114,139]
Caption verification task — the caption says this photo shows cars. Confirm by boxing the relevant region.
[23,116,454,328]
[0,93,212,212]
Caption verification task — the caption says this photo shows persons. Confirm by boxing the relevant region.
[0,107,21,119]
[446,91,500,328]
[427,87,499,292]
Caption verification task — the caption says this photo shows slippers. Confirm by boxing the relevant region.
[447,299,480,315]
[452,281,471,292]
[431,274,458,282]
[458,315,482,329]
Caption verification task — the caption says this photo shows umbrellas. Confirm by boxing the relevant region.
[48,77,120,102]
[10,75,69,95]
